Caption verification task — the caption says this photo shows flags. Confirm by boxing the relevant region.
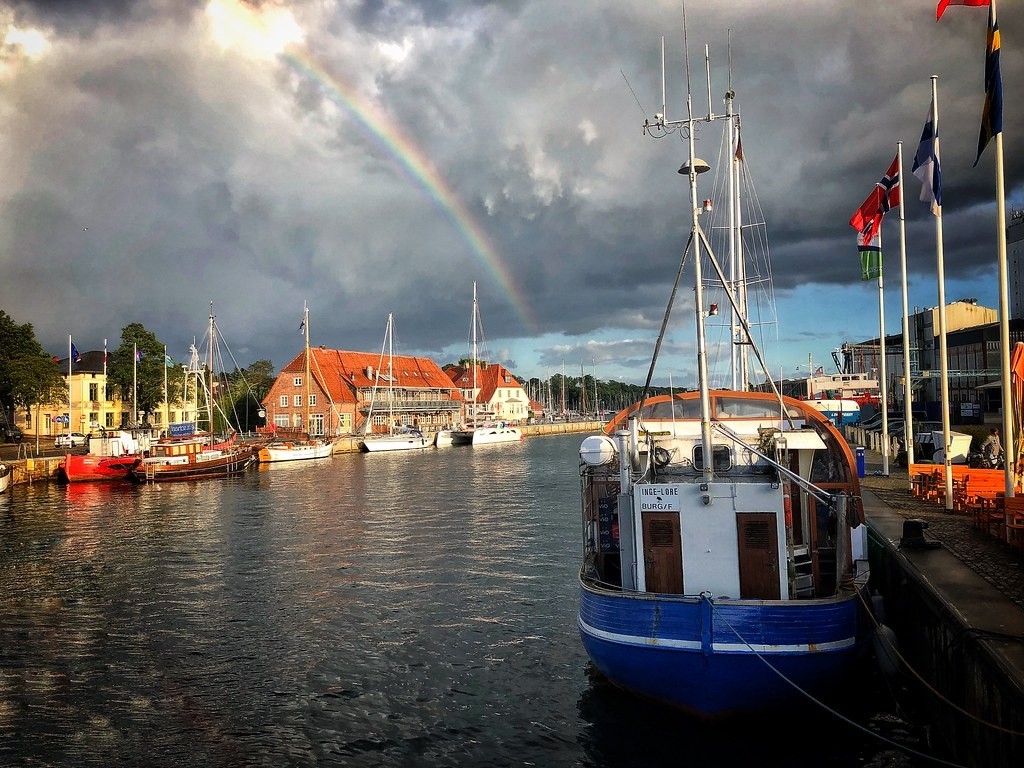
[167,354,174,368]
[936,0,1003,167]
[911,104,941,216]
[849,153,899,246]
[858,232,880,281]
[71,342,81,364]
[136,350,143,364]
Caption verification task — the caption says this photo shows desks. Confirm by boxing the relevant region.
[976,493,1004,536]
[917,471,933,500]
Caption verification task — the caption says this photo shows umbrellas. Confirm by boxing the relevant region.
[1010,342,1024,487]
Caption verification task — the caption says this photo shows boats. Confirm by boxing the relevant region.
[0,463,19,494]
[59,451,141,484]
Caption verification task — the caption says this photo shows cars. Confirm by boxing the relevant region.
[0,423,24,444]
[54,431,86,449]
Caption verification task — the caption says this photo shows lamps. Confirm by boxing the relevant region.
[771,482,780,489]
[700,484,708,491]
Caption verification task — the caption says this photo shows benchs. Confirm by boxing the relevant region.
[910,464,1024,553]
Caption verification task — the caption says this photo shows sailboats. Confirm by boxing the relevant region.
[248,297,353,463]
[129,297,280,484]
[435,278,526,449]
[350,309,432,452]
[572,0,873,720]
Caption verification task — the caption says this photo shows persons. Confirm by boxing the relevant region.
[84,431,92,446]
[980,428,1003,469]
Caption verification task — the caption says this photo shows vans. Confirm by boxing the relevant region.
[855,410,944,464]
